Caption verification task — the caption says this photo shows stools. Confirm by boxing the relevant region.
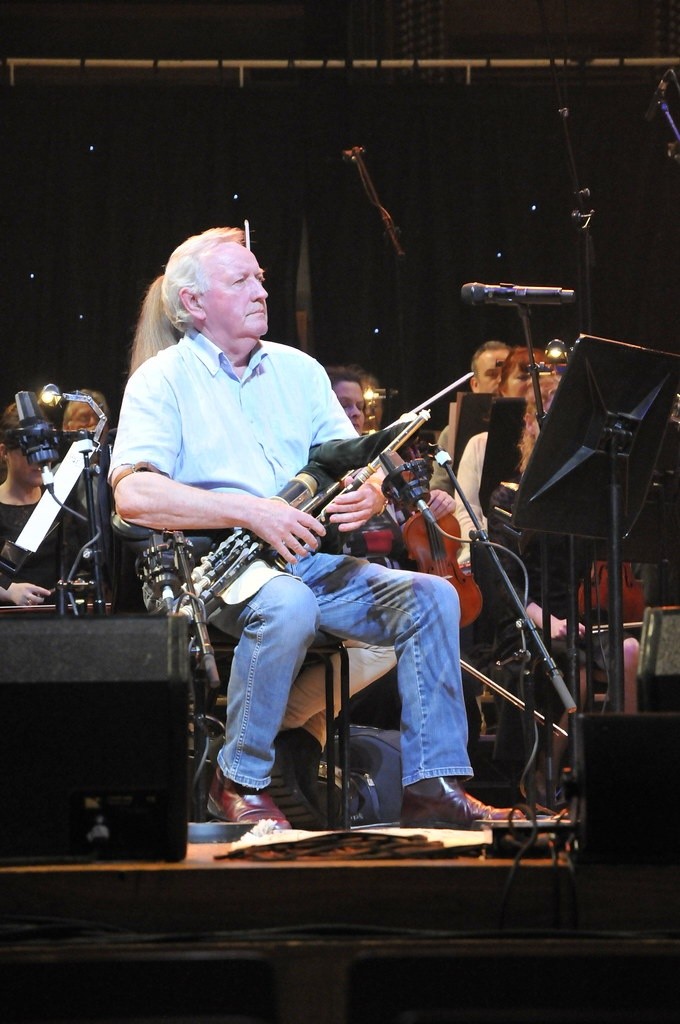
[110,508,351,831]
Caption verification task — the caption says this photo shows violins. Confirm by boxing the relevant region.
[576,535,646,633]
[403,438,484,630]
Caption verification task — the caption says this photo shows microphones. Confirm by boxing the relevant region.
[380,451,437,524]
[645,69,674,122]
[461,282,573,307]
[15,391,56,494]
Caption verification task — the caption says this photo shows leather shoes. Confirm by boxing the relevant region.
[399,787,526,830]
[208,766,292,830]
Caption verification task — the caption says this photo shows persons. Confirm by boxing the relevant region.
[324,341,639,817]
[106,227,525,830]
[0,387,111,606]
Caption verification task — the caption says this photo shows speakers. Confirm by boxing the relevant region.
[571,714,680,868]
[637,605,680,715]
[0,609,189,863]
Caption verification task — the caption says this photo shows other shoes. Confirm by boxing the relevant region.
[264,728,329,831]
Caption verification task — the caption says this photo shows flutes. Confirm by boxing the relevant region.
[171,406,432,621]
